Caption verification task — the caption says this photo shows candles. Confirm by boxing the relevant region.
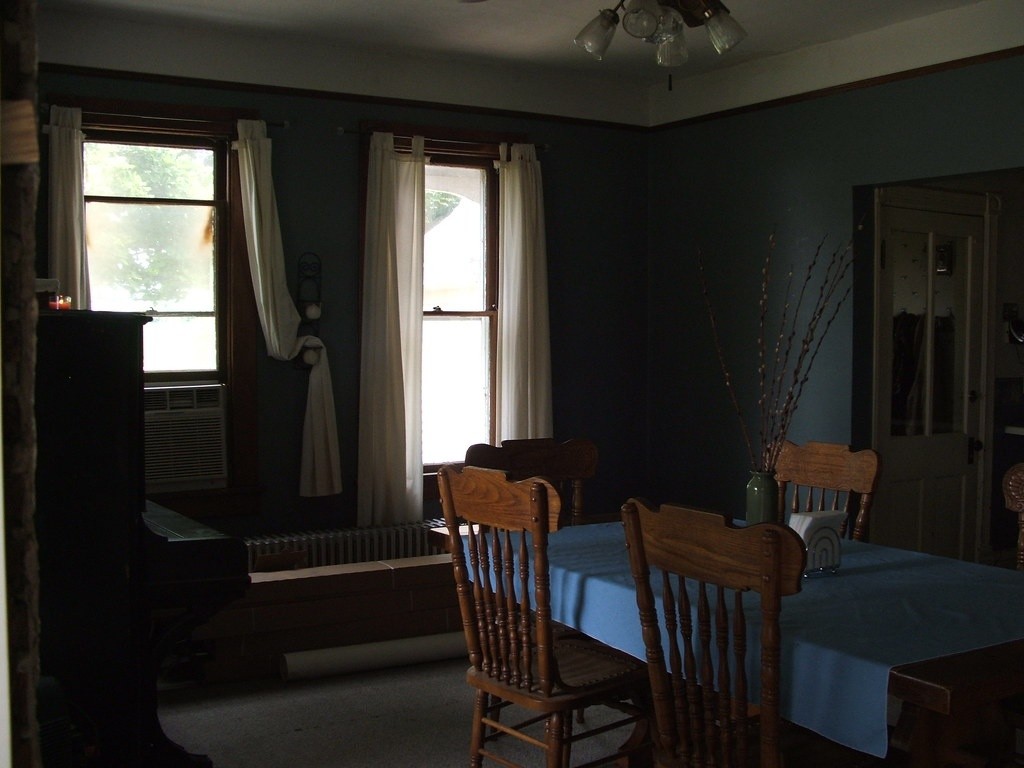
[50,295,72,310]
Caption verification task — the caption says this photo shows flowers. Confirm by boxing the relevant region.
[696,219,861,473]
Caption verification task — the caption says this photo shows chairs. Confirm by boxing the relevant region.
[464,437,597,740]
[621,498,806,768]
[778,439,882,543]
[437,465,650,768]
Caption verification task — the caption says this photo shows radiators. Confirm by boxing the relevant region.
[243,518,451,572]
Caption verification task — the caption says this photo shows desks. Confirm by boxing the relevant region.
[460,518,1024,768]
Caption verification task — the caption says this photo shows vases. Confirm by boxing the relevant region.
[744,469,778,527]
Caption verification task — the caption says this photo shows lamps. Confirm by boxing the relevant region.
[574,0,749,68]
[935,241,958,274]
